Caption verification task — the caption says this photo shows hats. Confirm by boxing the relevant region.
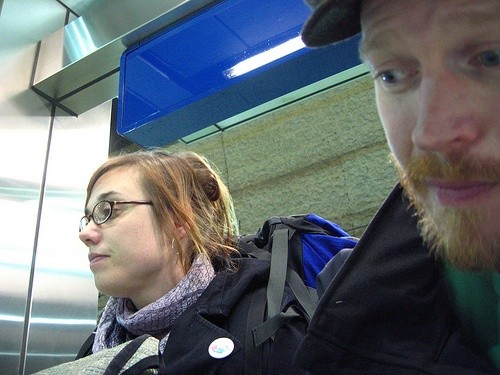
[302,0,363,47]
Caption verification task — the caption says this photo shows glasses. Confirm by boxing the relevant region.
[77,199,153,234]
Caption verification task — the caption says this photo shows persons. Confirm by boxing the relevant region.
[75,148,325,375]
[288,0,500,375]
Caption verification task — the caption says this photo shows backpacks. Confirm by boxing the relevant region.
[231,214,362,330]
